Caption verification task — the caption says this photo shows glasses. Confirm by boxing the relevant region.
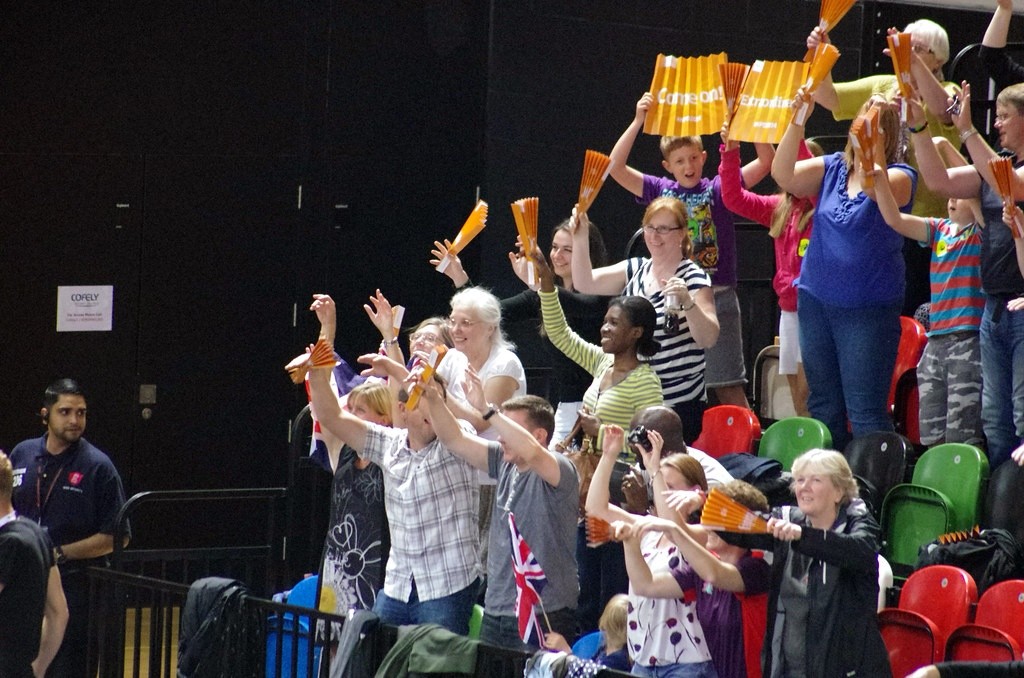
[446,317,483,330]
[643,222,682,235]
[409,332,447,345]
[995,111,1024,124]
[911,45,936,57]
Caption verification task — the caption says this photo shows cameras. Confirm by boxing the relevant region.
[628,425,653,452]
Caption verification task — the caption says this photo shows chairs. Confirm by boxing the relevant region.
[263,307,1024,678]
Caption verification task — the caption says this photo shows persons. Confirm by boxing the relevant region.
[429,0,1024,466]
[9,378,131,678]
[0,450,69,678]
[305,235,1023,678]
[909,121,928,134]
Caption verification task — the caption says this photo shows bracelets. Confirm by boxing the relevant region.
[384,337,399,347]
[960,128,978,143]
[681,298,695,312]
[321,333,335,341]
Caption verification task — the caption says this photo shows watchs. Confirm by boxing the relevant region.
[55,547,68,563]
[483,403,499,420]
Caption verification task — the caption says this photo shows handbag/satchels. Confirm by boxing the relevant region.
[915,528,1021,604]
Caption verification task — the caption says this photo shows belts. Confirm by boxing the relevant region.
[991,291,1024,324]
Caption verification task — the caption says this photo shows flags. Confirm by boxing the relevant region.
[508,514,546,644]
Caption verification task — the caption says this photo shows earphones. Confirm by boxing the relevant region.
[42,412,49,421]
